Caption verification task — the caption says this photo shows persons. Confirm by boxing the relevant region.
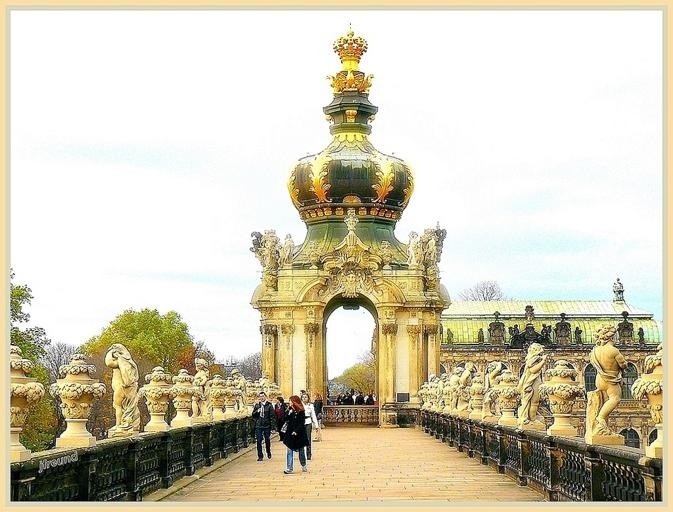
[327,388,377,405]
[517,343,545,422]
[232,367,248,413]
[427,361,503,420]
[260,367,270,399]
[588,324,628,435]
[106,345,140,431]
[251,388,324,474]
[190,357,212,420]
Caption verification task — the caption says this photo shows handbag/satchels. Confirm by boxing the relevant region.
[280,422,288,433]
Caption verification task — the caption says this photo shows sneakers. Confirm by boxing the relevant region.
[257,454,272,461]
[284,468,294,474]
[301,466,307,472]
